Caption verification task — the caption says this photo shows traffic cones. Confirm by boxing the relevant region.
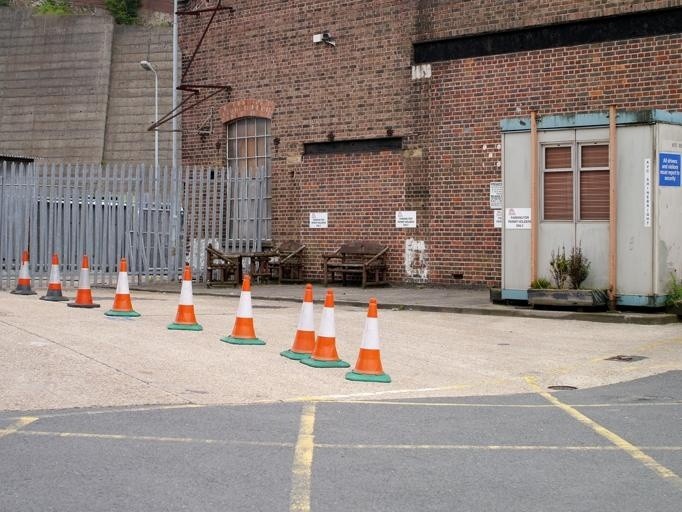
[280,281,318,361]
[344,295,394,383]
[218,274,267,345]
[40,252,70,302]
[105,255,142,318]
[67,255,104,309]
[167,263,204,332]
[9,248,37,296]
[297,288,353,370]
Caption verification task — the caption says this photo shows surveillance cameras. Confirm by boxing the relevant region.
[323,31,331,40]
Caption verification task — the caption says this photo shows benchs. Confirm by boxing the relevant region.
[321,239,390,290]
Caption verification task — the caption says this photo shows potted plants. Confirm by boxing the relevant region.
[527,242,608,307]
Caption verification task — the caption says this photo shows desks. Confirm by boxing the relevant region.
[237,250,279,283]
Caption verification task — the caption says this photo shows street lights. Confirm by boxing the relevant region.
[140,58,161,213]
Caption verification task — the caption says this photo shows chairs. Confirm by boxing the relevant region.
[207,245,240,288]
[272,240,307,282]
